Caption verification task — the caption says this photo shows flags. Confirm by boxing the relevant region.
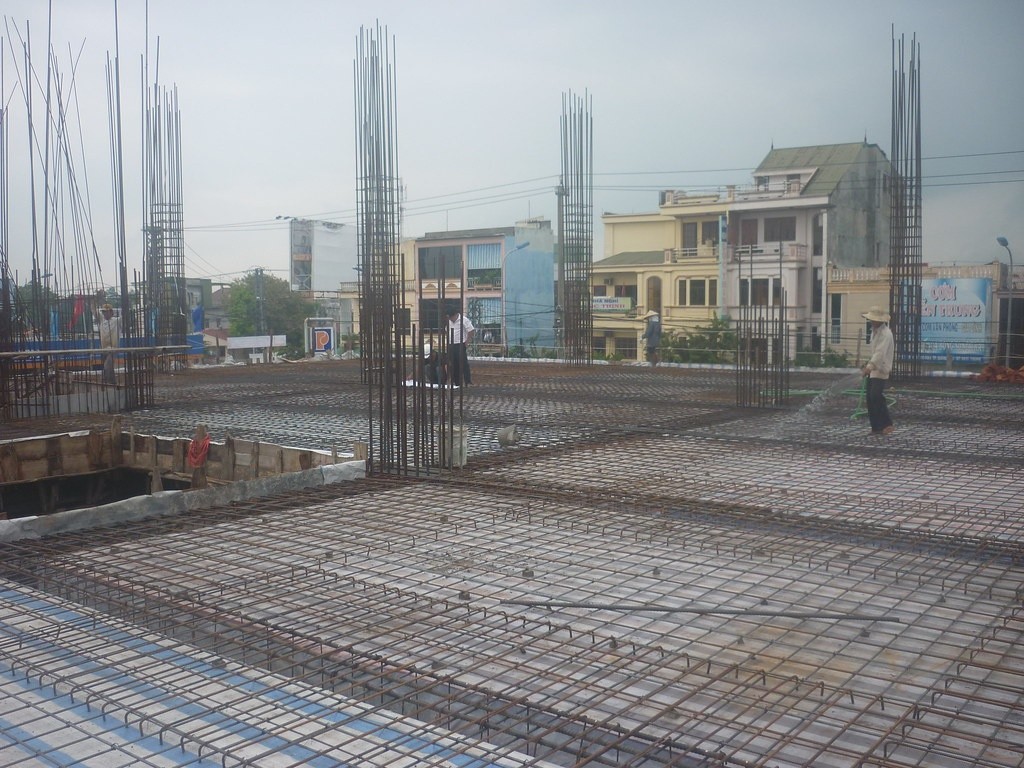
[192,302,204,333]
[66,293,84,332]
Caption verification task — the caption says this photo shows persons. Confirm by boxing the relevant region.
[859,308,895,438]
[89,296,124,385]
[405,342,449,385]
[640,309,662,371]
[443,308,475,387]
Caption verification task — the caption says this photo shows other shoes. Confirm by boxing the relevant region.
[466,383,474,387]
[870,425,896,435]
[652,359,659,367]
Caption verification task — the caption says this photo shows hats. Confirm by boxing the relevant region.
[862,305,891,323]
[424,343,431,359]
[100,303,113,310]
[644,310,659,320]
